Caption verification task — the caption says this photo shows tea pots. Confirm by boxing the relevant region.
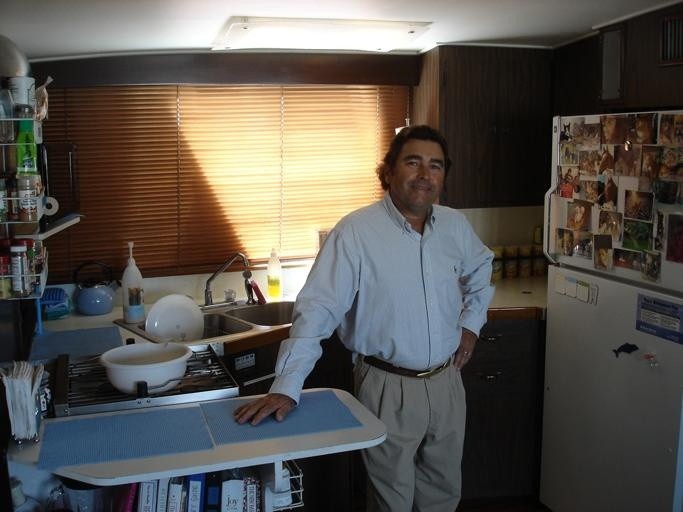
[73,261,122,316]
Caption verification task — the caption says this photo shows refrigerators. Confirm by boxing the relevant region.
[539,109,682,512]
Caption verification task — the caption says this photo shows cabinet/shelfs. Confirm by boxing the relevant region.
[550,39,600,114]
[412,47,551,213]
[464,317,540,500]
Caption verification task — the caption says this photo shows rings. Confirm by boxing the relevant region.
[464,352,468,354]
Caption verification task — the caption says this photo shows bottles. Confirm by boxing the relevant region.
[0,77,47,299]
[9,476,25,506]
[486,244,541,281]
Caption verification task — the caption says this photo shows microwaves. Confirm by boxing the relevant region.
[35,142,80,234]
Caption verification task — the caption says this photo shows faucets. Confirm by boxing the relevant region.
[206,253,248,290]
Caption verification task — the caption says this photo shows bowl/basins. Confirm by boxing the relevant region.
[100,294,205,393]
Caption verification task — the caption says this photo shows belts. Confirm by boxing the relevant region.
[363,355,450,378]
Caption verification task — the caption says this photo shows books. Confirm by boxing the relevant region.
[122,477,186,512]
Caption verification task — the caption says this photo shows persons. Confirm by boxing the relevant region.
[592,244,611,270]
[233,124,496,512]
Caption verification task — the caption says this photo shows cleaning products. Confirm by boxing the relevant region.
[267,248,281,299]
[123,242,146,324]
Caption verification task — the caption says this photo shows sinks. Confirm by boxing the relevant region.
[112,314,252,345]
[226,302,295,326]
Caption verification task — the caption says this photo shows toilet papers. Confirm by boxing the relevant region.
[44,196,60,216]
